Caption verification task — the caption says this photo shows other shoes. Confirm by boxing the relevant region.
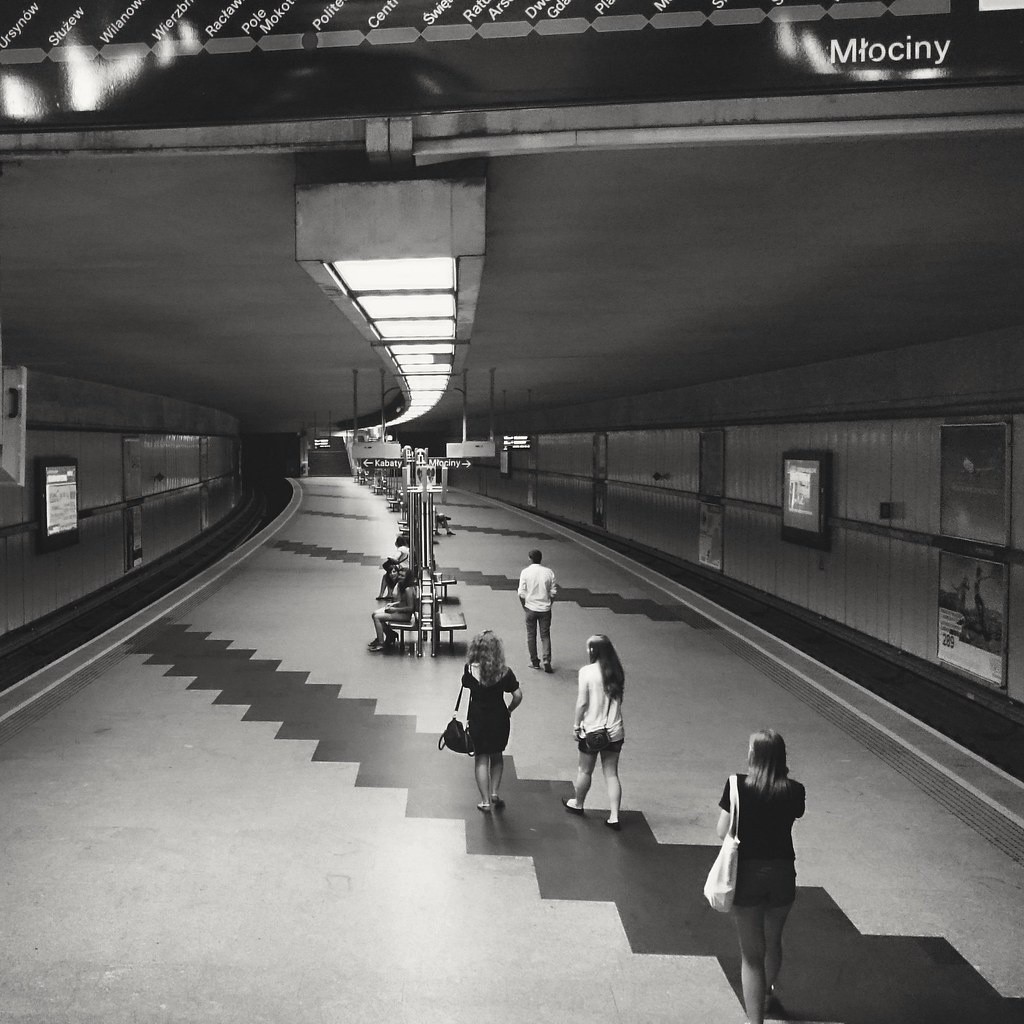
[477,801,491,812]
[491,793,503,805]
[370,637,382,647]
[562,797,584,815]
[368,645,383,651]
[603,818,621,830]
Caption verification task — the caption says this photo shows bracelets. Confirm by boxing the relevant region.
[508,705,514,712]
[572,725,581,729]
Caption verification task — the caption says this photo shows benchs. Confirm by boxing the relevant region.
[354,473,468,653]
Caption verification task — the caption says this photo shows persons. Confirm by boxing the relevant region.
[560,633,626,832]
[460,629,523,814]
[376,564,401,648]
[367,500,457,602]
[714,727,807,1024]
[516,549,558,674]
[943,561,997,642]
[368,567,415,652]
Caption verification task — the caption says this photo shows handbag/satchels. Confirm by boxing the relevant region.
[703,771,742,913]
[437,719,476,757]
[447,531,455,535]
[584,728,612,752]
[434,531,441,535]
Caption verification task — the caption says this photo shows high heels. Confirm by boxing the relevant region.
[393,629,399,642]
[384,628,395,645]
